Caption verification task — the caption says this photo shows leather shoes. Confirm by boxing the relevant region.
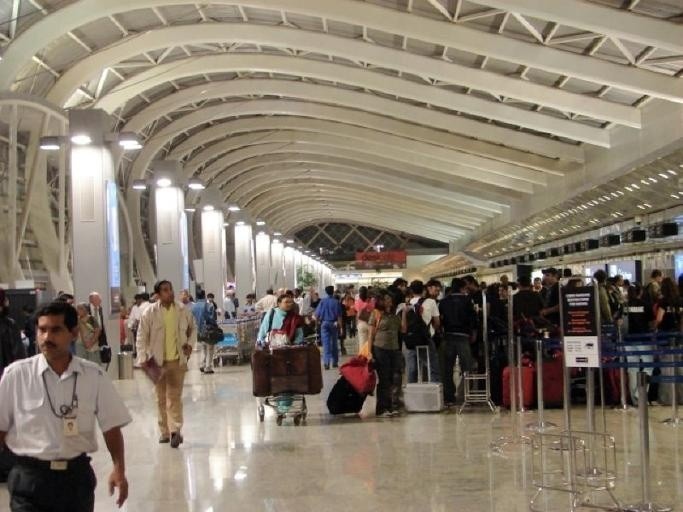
[171,431,180,447]
[159,433,170,444]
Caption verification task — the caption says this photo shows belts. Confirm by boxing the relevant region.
[21,458,87,474]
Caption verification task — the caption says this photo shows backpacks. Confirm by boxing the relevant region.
[404,298,433,350]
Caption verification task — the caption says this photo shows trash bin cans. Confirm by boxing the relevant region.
[118,351,133,379]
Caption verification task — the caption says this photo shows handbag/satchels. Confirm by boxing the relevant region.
[339,355,377,396]
[357,310,382,360]
[200,317,225,344]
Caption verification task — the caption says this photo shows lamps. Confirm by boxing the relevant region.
[40,127,241,227]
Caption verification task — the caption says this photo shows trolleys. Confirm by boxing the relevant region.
[214,312,260,367]
[258,346,307,425]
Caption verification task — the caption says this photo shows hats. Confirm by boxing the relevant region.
[541,268,557,275]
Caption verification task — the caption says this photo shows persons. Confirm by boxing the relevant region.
[368,269,683,420]
[278,286,322,346]
[311,285,344,370]
[206,293,217,322]
[191,284,217,373]
[243,293,258,317]
[335,290,341,296]
[254,287,277,313]
[257,293,304,414]
[223,289,239,318]
[0,301,132,512]
[334,294,347,356]
[0,290,112,376]
[136,280,197,447]
[120,292,159,358]
[355,286,376,351]
[342,284,357,338]
[178,288,194,311]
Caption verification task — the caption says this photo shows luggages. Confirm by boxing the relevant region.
[249,339,324,398]
[501,357,534,411]
[401,344,444,413]
[540,353,565,409]
[601,339,629,405]
[322,376,365,415]
